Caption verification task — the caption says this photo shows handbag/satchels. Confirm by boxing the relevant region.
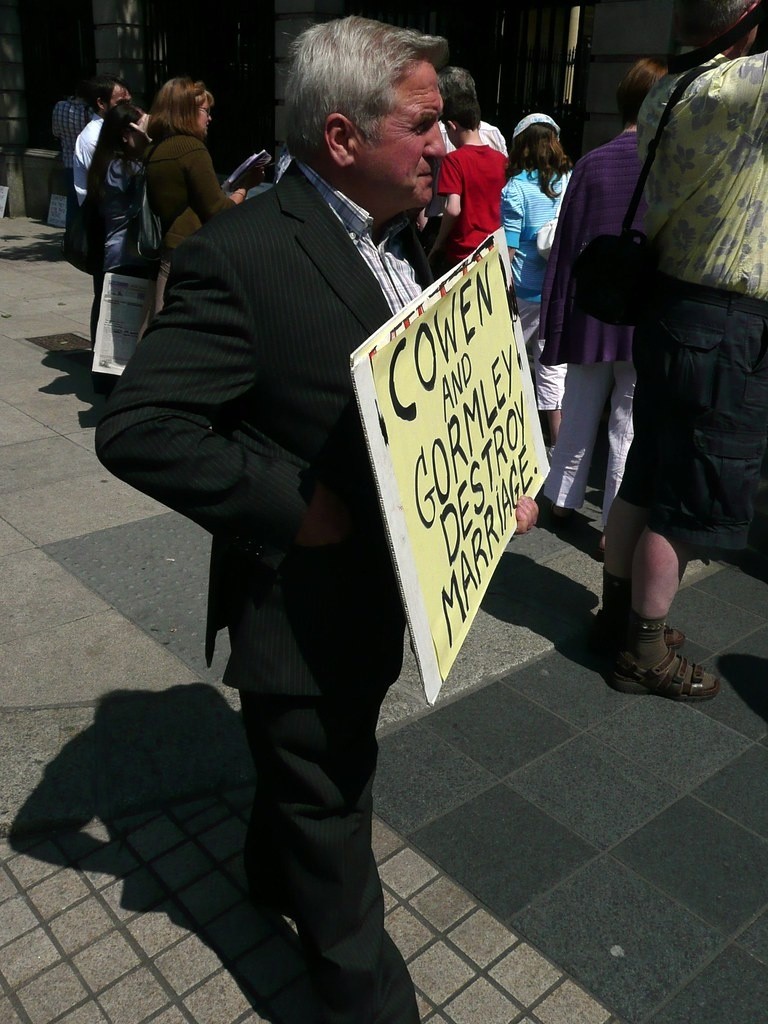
[136,177,171,260]
[537,217,558,261]
[573,230,660,326]
[62,195,97,275]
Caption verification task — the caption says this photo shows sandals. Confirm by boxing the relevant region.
[596,609,685,650]
[613,646,720,700]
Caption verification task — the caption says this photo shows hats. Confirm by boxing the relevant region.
[513,113,560,142]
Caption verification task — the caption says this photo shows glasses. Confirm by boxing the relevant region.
[196,104,211,115]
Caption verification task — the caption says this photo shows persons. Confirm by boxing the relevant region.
[539,56,685,550]
[603,4,768,703]
[501,114,588,454]
[72,18,541,1024]
